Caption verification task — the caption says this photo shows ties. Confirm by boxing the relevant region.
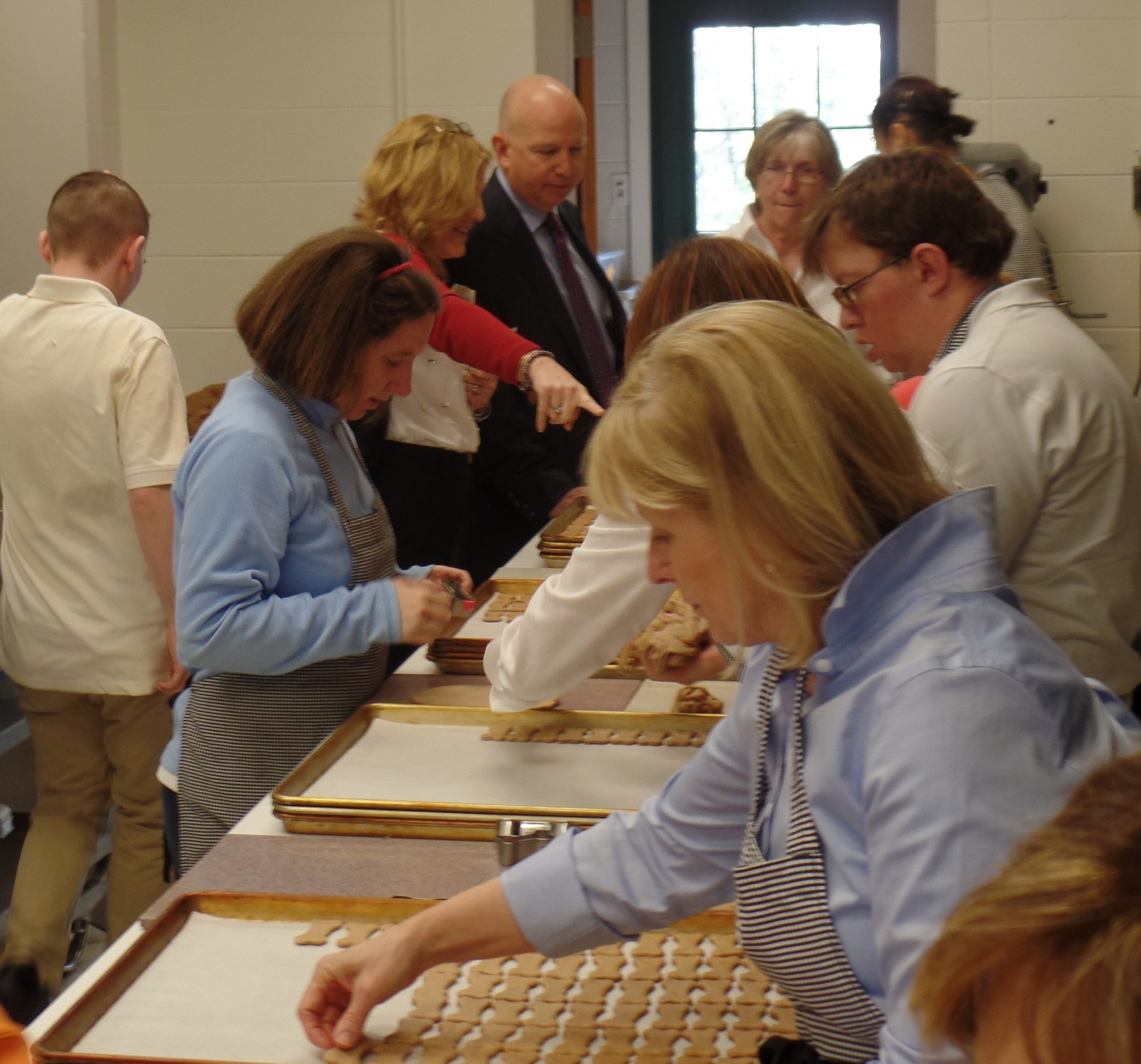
[542,215,620,410]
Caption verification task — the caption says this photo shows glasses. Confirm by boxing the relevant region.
[833,248,956,309]
[759,165,826,185]
[416,118,475,149]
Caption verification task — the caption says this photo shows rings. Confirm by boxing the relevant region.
[471,385,478,394]
[551,405,564,414]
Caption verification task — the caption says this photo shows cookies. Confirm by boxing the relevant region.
[483,593,530,624]
[480,723,708,747]
[293,918,798,1064]
[556,508,598,537]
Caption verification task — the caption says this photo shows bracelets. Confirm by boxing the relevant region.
[515,348,555,392]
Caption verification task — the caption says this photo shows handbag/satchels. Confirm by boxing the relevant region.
[385,343,481,453]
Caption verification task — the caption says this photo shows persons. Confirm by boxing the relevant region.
[339,110,604,594]
[0,171,189,1030]
[719,108,905,388]
[295,299,1141,1064]
[157,229,474,879]
[484,227,821,713]
[869,78,1062,308]
[798,145,1141,724]
[433,71,634,550]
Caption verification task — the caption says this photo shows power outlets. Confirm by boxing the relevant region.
[613,174,629,204]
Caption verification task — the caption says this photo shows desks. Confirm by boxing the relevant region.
[28,521,739,1064]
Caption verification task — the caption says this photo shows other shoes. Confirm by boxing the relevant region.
[0,962,46,1026]
